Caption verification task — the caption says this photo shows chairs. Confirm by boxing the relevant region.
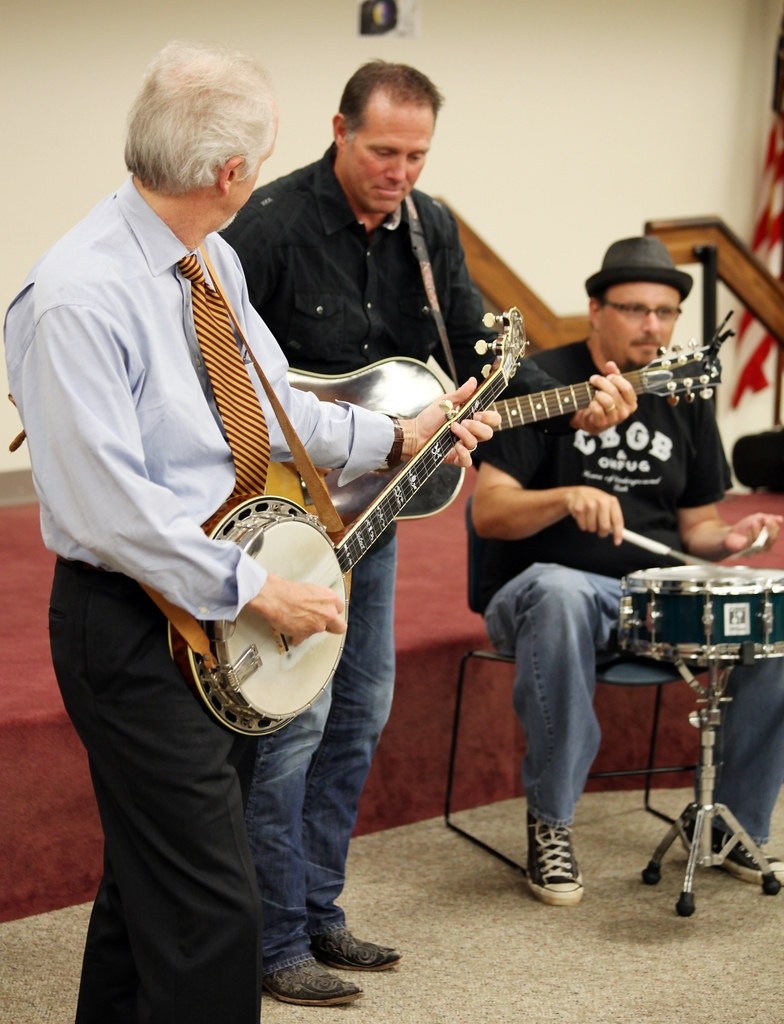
[439,497,733,875]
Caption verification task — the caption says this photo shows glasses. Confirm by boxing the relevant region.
[527,810,583,905]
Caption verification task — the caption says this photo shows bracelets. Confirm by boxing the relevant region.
[376,411,404,472]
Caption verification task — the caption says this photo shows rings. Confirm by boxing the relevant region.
[605,403,615,413]
[469,445,477,452]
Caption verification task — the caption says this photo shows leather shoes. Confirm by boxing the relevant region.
[263,959,365,1005]
[308,928,404,971]
[586,236,695,303]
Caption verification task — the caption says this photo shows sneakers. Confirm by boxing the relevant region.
[685,819,784,887]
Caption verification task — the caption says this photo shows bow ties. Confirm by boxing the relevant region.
[597,296,681,321]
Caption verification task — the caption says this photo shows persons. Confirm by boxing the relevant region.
[5,40,504,1024]
[472,236,784,906]
[214,64,637,1008]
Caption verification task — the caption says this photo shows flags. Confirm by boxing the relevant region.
[731,21,784,406]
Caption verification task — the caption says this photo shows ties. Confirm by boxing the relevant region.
[176,254,276,500]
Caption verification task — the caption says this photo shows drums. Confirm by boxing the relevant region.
[616,563,784,668]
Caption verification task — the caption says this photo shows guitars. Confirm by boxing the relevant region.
[283,341,726,535]
[167,307,531,737]
